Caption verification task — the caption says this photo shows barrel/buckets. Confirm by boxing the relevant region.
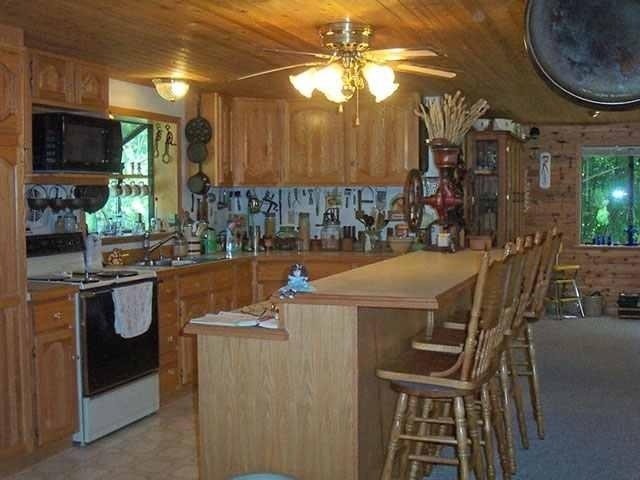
[583,296,604,317]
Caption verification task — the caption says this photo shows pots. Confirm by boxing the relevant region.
[186,141,210,195]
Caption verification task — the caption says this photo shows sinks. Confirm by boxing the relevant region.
[173,257,229,261]
[129,260,196,268]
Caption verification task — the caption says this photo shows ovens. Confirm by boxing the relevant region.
[79,276,161,395]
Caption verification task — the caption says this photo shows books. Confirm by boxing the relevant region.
[189,310,259,327]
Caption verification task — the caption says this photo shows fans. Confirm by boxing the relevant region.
[238,21,456,110]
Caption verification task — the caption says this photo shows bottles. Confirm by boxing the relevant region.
[134,213,166,233]
[208,227,217,254]
[103,215,123,235]
[55,207,76,234]
[320,225,341,252]
[265,212,311,251]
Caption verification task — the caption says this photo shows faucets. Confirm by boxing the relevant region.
[143,231,182,257]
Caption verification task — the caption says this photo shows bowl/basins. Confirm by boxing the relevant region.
[388,238,412,253]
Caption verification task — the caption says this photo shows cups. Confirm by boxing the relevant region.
[436,232,451,247]
[472,117,514,132]
[342,226,356,240]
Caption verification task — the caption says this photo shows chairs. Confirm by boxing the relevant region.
[545,231,586,319]
[375,227,564,479]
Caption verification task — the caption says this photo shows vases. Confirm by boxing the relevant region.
[431,145,459,168]
[467,233,494,250]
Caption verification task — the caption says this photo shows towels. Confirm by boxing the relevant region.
[111,281,155,339]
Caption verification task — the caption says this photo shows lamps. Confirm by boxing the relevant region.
[286,54,399,107]
[151,79,192,104]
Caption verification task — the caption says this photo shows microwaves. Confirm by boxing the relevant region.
[30,107,122,173]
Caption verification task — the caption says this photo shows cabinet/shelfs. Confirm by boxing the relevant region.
[32,55,109,113]
[189,93,283,186]
[465,130,527,248]
[159,260,253,406]
[285,95,419,185]
[257,260,356,300]
[33,295,82,462]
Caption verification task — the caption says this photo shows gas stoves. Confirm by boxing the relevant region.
[26,269,156,285]
